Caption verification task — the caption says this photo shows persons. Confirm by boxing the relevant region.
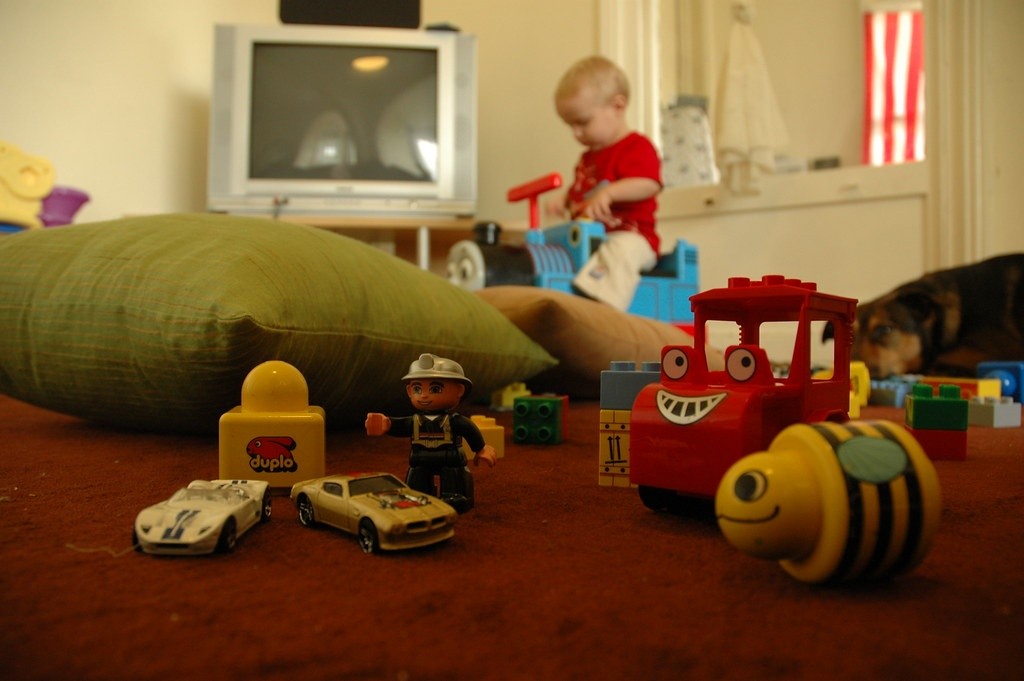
[554,56,666,313]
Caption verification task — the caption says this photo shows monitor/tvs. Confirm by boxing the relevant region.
[205,25,477,221]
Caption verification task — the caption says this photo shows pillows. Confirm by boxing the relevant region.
[481,285,724,397]
[0,211,559,431]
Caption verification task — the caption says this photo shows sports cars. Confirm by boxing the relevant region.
[134,480,271,555]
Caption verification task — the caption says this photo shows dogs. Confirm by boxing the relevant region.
[822,253,1024,380]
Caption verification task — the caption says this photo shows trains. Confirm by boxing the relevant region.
[444,172,699,323]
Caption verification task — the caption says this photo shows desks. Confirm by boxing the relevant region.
[280,217,502,274]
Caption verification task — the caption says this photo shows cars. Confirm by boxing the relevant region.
[631,275,858,510]
[290,472,457,553]
